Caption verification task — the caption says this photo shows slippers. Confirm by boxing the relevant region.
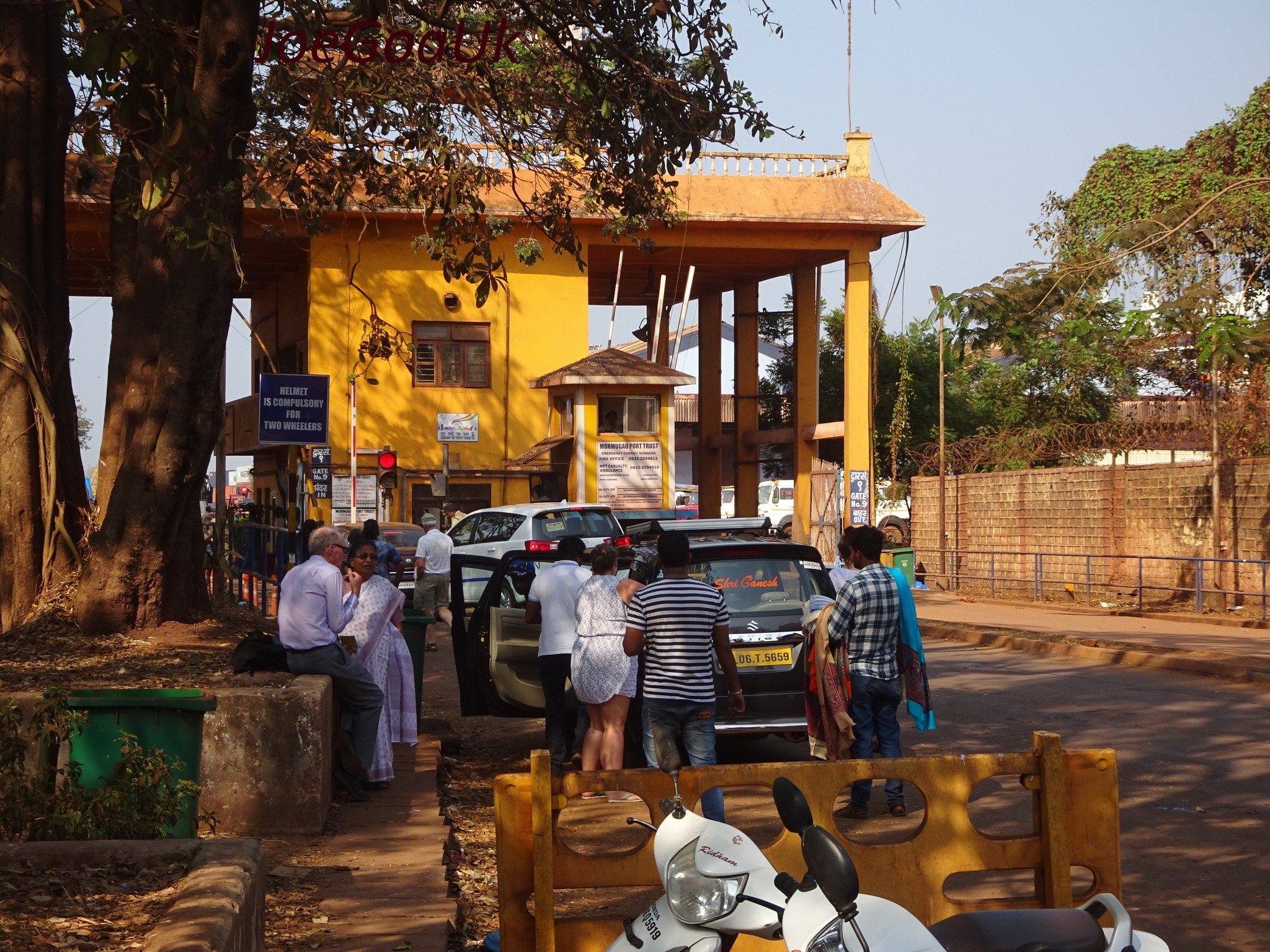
[581,791,608,800]
[609,794,643,802]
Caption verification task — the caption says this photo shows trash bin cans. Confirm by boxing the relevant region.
[891,547,915,587]
[880,549,896,568]
[43,683,215,839]
[399,605,435,732]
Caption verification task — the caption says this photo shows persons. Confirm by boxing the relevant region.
[415,512,454,652]
[622,531,745,831]
[815,524,936,820]
[569,542,646,804]
[599,411,623,433]
[339,538,420,791]
[200,499,469,591]
[828,533,864,596]
[524,536,596,771]
[275,528,384,803]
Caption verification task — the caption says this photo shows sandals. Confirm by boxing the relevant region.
[891,805,906,816]
[425,642,438,651]
[833,802,868,819]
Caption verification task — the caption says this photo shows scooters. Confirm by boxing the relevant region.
[603,720,1170,951]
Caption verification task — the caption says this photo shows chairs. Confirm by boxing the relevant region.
[723,590,809,613]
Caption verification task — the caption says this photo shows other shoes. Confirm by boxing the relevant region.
[333,759,371,801]
[333,749,346,794]
[367,781,392,790]
[571,753,583,771]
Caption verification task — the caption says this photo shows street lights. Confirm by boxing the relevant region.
[1192,226,1226,613]
[931,284,947,576]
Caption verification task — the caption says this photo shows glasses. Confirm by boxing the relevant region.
[327,544,348,554]
[444,512,449,516]
[353,553,378,561]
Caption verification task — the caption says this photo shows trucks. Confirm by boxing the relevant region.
[756,480,911,548]
[720,488,735,518]
[674,490,699,522]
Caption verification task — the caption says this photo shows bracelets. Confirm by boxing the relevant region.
[727,689,742,695]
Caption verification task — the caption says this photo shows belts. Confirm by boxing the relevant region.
[286,645,329,653]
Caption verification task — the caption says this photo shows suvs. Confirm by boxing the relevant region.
[450,515,837,765]
[334,519,427,607]
[444,499,630,605]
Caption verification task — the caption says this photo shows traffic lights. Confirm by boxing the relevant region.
[378,449,398,488]
